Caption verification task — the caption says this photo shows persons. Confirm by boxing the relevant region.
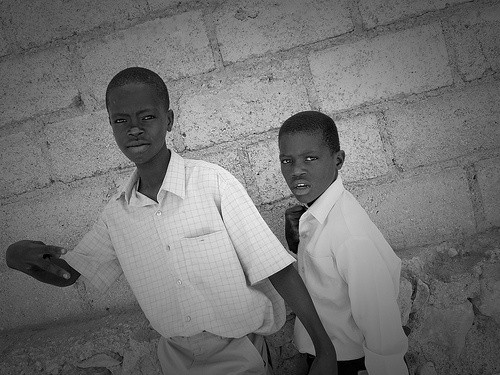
[5,67,336,375]
[277,110,412,375]
